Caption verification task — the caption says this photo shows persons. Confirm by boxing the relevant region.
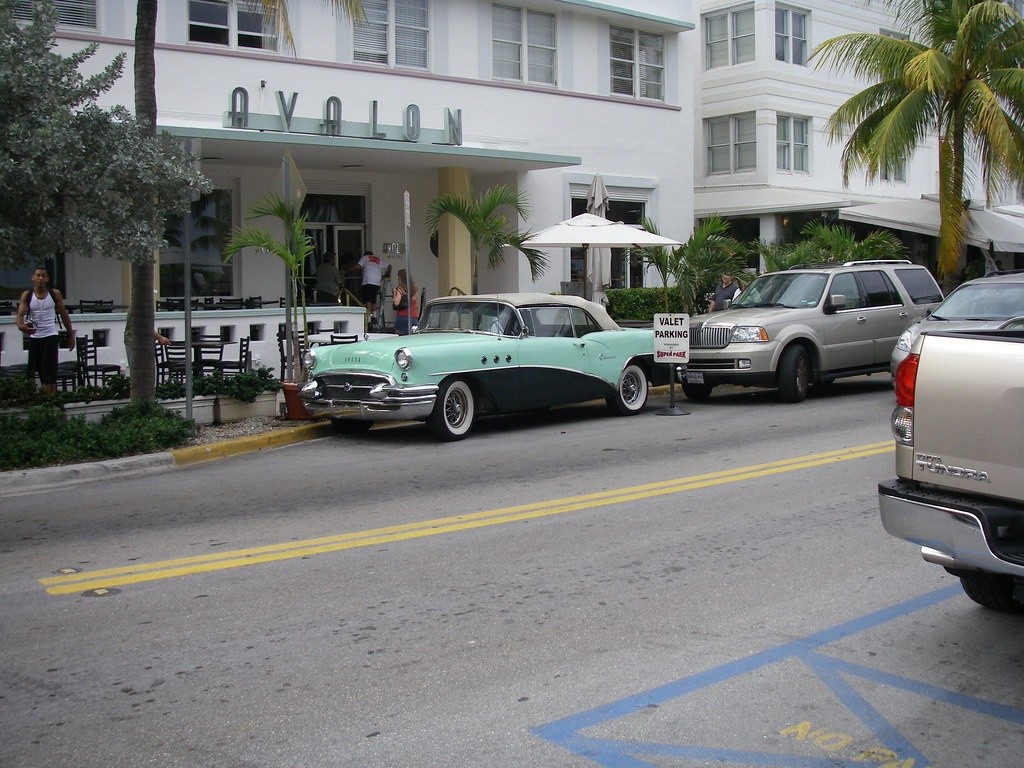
[709,268,761,312]
[17,266,75,397]
[343,251,382,329]
[123,287,171,405]
[393,269,418,336]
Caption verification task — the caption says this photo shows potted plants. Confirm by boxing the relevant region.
[222,194,321,419]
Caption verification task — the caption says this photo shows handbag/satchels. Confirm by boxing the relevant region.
[392,287,409,310]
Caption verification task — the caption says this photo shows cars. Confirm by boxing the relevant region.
[296,291,672,443]
[880,267,1024,614]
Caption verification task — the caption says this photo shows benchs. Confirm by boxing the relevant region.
[535,325,599,338]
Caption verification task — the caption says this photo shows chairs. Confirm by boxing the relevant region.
[155,334,224,387]
[217,336,249,374]
[0,332,121,392]
[79,299,114,314]
[276,323,358,383]
[0,301,18,316]
[156,296,311,343]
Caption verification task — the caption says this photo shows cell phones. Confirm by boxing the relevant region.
[27,322,33,328]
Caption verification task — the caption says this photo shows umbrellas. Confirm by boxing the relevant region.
[498,212,683,301]
[586,174,613,309]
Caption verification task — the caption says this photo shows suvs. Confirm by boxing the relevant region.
[675,259,946,404]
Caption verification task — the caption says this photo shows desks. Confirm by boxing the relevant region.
[191,303,241,309]
[262,301,279,304]
[160,341,237,376]
[63,305,128,311]
[307,333,399,348]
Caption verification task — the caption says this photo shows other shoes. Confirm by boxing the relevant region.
[368,322,373,330]
[372,317,378,324]
